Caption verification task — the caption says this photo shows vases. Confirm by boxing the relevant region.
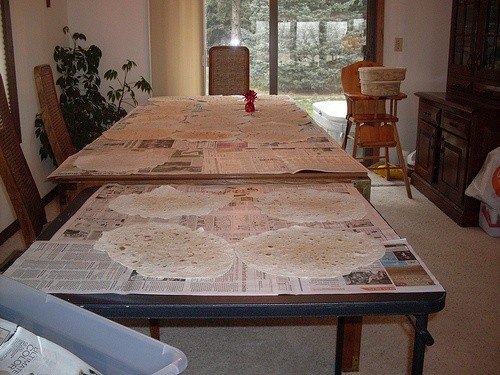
[244,102,256,113]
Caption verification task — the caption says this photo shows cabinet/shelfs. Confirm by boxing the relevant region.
[411,0,500,227]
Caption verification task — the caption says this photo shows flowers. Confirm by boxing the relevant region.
[242,91,257,101]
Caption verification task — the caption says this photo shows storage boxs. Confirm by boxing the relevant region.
[0,274,189,375]
[357,67,408,81]
[359,80,400,97]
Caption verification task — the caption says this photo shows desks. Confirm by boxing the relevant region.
[0,182,446,375]
[46,95,371,202]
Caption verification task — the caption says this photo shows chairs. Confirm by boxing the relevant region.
[341,61,413,199]
[14,127,47,249]
[33,64,77,167]
[208,46,250,95]
